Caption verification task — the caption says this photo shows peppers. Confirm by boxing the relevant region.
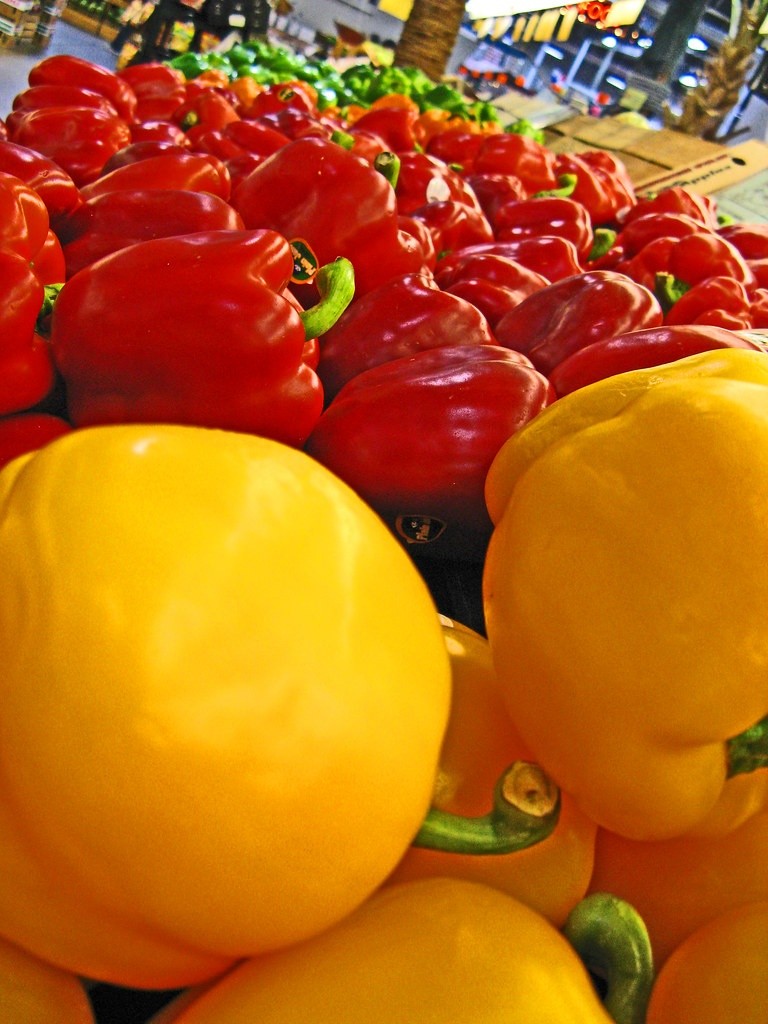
[0,37,768,1024]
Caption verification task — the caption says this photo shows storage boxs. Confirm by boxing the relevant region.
[0,0,68,52]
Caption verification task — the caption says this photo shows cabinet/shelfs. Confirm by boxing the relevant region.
[60,0,221,60]
